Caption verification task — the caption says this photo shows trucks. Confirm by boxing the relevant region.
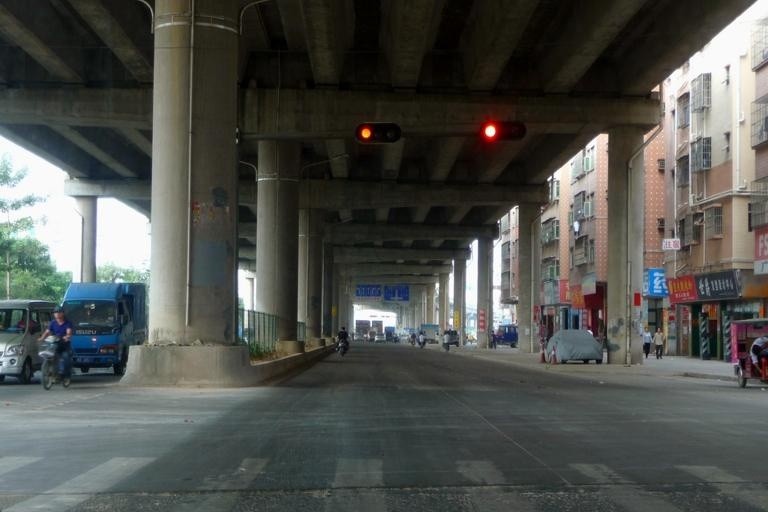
[496,324,518,347]
[420,324,439,344]
[385,326,395,341]
[58,282,146,374]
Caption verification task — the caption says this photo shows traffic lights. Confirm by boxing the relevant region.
[482,122,526,141]
[354,121,403,144]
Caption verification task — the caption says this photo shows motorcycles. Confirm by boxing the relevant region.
[336,339,348,357]
[407,337,426,348]
[38,335,71,391]
[730,318,768,387]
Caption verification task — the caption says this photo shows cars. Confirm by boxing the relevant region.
[442,329,459,347]
[367,330,384,343]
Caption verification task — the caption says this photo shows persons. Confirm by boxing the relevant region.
[443,331,449,351]
[410,331,427,349]
[643,327,652,359]
[490,330,496,349]
[105,312,115,323]
[335,326,349,352]
[749,337,768,376]
[17,311,37,334]
[37,305,74,379]
[652,327,666,359]
[586,326,593,335]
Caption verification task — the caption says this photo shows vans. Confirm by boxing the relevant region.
[0,299,57,384]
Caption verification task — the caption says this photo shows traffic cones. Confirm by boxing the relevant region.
[539,349,546,363]
[551,346,557,365]
